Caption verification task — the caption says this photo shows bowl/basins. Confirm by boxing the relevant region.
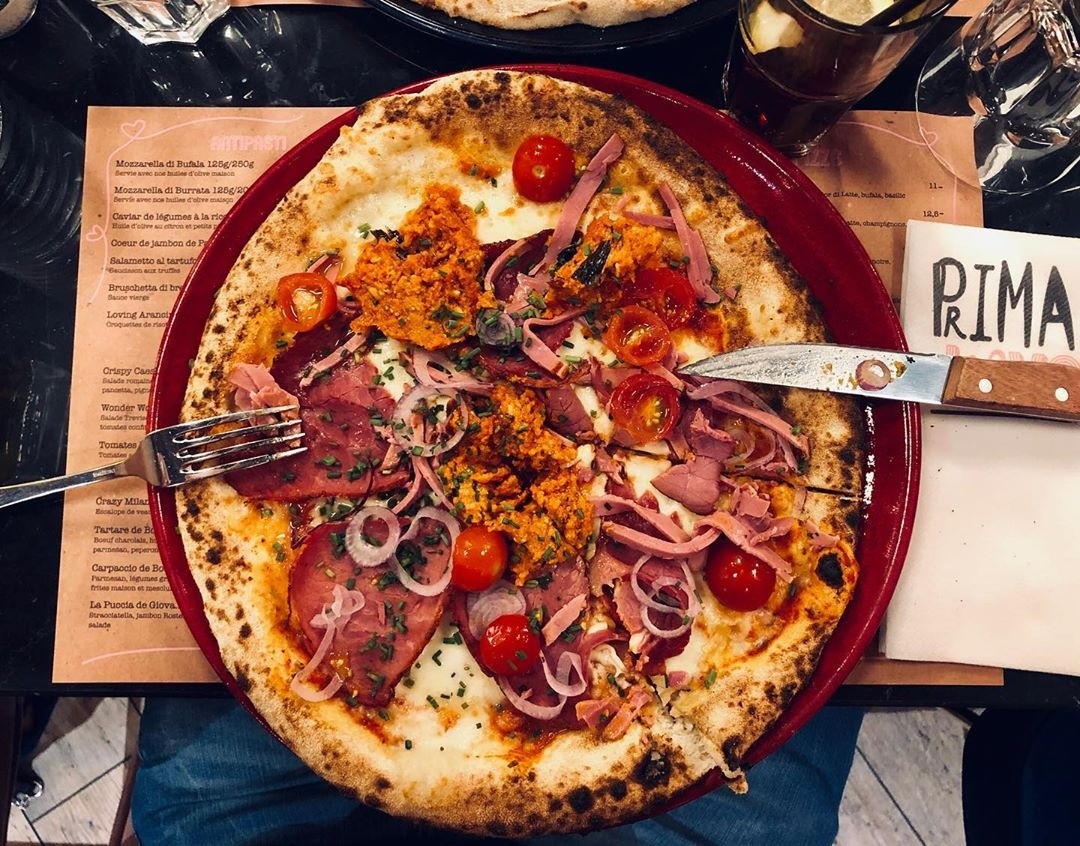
[0,0,38,37]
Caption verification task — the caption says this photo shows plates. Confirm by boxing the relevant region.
[143,64,923,821]
[368,0,742,57]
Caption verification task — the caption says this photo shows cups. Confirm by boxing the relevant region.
[911,1,1080,197]
[87,0,233,47]
[720,0,963,161]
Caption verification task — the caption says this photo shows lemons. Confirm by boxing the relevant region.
[747,0,900,53]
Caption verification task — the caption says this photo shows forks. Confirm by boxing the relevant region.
[1,405,311,515]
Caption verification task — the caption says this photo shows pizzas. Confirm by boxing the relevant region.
[175,70,861,838]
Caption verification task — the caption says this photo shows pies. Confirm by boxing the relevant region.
[412,0,694,29]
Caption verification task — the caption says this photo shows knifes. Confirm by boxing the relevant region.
[673,343,1079,423]
